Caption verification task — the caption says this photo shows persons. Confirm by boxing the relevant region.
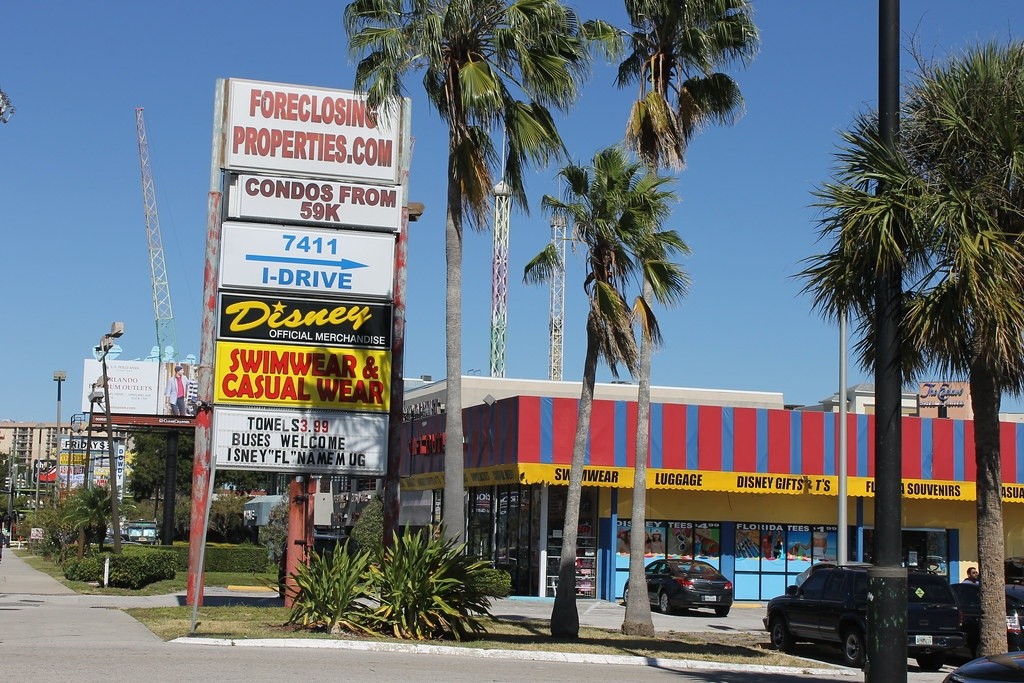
[616,527,664,557]
[763,530,827,560]
[164,364,192,417]
[961,567,979,585]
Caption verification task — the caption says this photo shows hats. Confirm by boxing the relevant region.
[652,529,662,535]
[175,365,183,373]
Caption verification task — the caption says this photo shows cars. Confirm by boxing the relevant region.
[977,556,1024,584]
[950,580,1024,659]
[624,559,734,616]
[795,561,873,588]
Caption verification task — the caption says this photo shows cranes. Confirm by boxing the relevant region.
[134,104,204,359]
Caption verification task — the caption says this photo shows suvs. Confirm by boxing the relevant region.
[761,564,966,671]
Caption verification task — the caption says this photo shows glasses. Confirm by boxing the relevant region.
[677,535,687,552]
